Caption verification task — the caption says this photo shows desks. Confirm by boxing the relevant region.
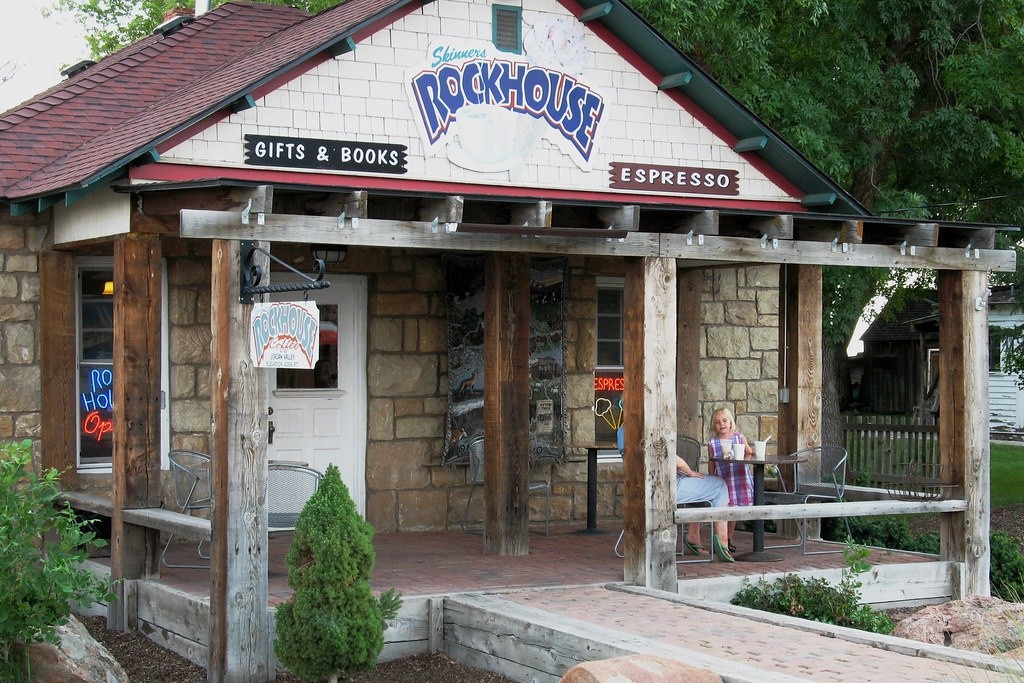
[709,455,808,561]
[556,441,619,536]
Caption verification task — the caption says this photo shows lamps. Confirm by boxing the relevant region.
[102,281,114,294]
[310,244,349,265]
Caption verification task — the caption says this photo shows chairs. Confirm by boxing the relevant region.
[763,446,855,556]
[614,435,714,564]
[160,450,212,569]
[461,437,550,537]
[268,461,325,575]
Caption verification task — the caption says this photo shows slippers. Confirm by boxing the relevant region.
[682,535,710,556]
[714,535,735,563]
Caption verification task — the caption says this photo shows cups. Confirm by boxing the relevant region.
[754,440,766,461]
[733,444,746,460]
[720,439,732,459]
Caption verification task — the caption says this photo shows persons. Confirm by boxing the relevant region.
[617,422,735,562]
[708,407,757,551]
